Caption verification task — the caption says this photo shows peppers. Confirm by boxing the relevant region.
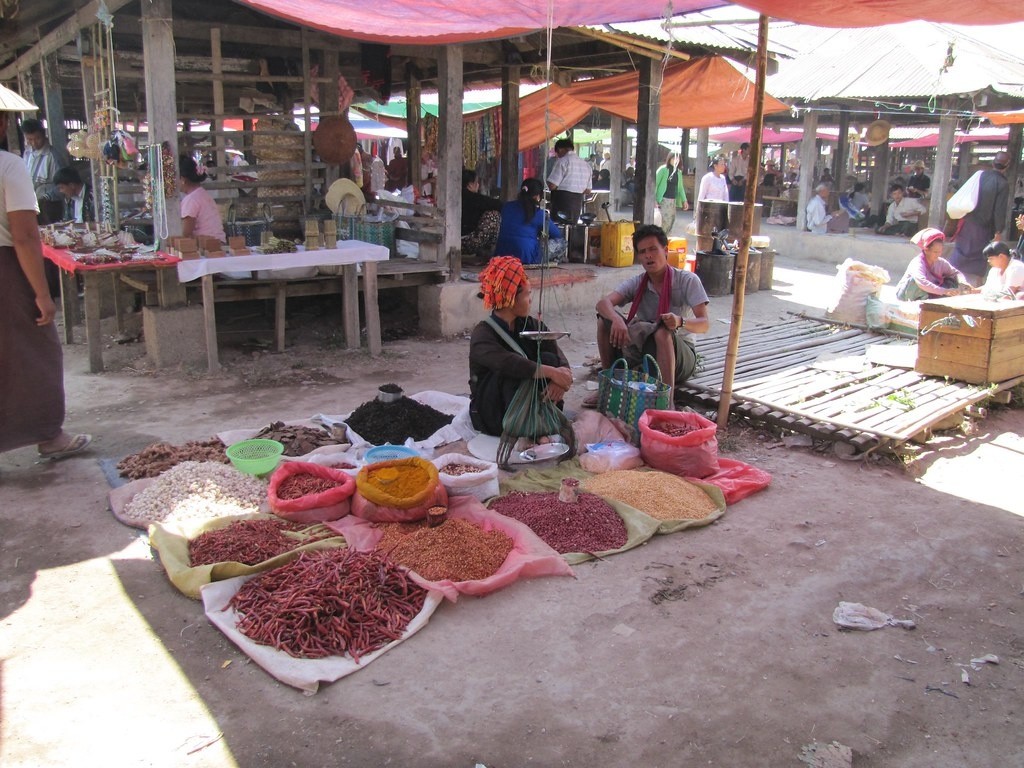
[277,472,342,500]
[649,422,705,438]
[189,516,341,566]
[216,545,427,663]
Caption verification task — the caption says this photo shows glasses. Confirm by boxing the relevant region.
[914,168,923,171]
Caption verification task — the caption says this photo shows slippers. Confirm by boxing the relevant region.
[37,433,92,458]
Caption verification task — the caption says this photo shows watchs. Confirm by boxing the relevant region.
[679,317,686,328]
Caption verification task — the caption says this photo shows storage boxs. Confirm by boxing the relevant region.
[914,294,1024,386]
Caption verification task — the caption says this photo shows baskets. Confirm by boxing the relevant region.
[227,204,274,248]
[337,215,395,260]
[597,354,672,446]
[253,118,307,227]
[365,445,419,464]
[225,439,284,475]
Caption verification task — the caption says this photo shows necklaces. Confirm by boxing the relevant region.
[145,144,169,240]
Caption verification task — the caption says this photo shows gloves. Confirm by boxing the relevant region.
[37,194,50,210]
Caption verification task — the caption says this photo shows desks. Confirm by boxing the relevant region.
[39,234,181,374]
[177,240,389,373]
[762,196,798,218]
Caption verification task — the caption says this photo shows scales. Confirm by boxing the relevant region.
[518,339,570,463]
[519,235,571,342]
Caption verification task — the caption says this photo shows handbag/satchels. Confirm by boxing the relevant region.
[502,374,574,438]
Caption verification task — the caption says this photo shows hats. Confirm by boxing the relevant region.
[225,147,244,155]
[313,117,357,164]
[325,179,367,214]
[911,160,927,168]
[866,121,891,147]
[995,151,1011,165]
[0,83,39,111]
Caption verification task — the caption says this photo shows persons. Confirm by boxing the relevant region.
[546,139,593,223]
[497,178,567,264]
[588,140,1024,306]
[179,154,226,246]
[0,84,93,461]
[19,120,65,298]
[578,224,710,412]
[470,256,573,451]
[460,169,502,260]
[388,146,408,190]
[52,168,95,222]
